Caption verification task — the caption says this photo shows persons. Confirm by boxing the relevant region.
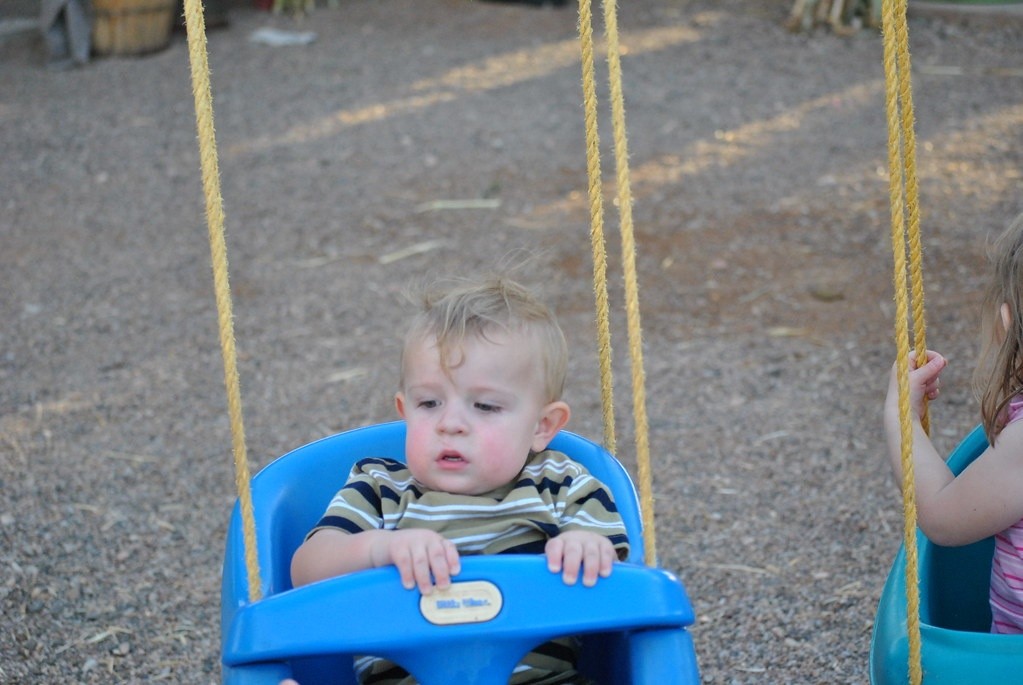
[290,277,629,685]
[884,214,1023,637]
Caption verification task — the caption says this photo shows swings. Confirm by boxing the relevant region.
[181,0,1022,685]
